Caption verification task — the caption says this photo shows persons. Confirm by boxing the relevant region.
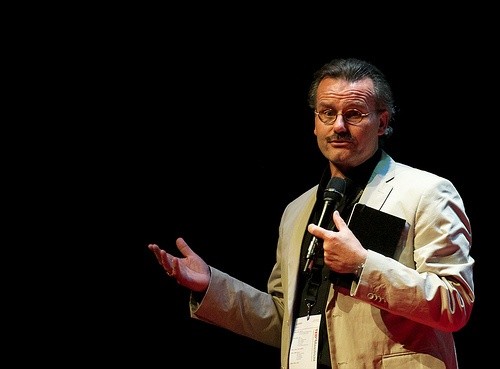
[148,57,475,369]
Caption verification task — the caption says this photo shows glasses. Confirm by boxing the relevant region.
[314,106,382,125]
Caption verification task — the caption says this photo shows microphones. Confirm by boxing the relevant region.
[302,176,346,272]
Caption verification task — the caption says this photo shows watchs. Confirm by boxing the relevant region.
[355,264,364,281]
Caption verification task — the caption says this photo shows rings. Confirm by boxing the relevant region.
[166,269,175,278]
